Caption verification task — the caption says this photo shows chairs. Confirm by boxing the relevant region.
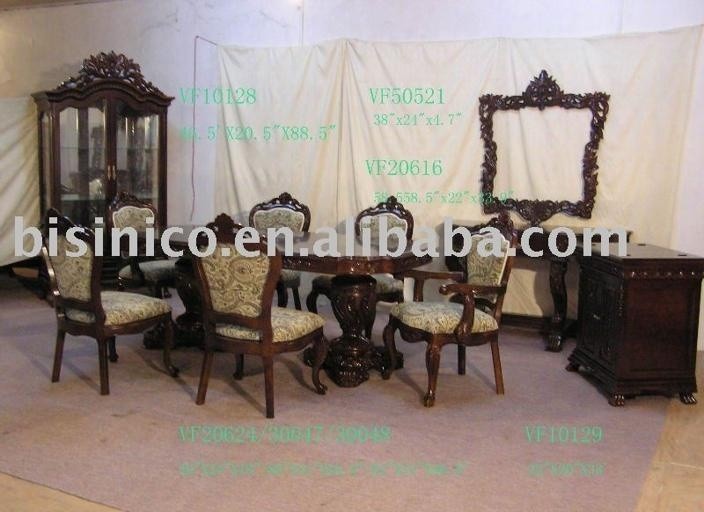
[381,216,519,408]
[249,191,311,312]
[302,195,416,380]
[37,207,181,396]
[106,190,186,335]
[192,213,329,419]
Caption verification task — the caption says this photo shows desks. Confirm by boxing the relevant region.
[444,219,631,352]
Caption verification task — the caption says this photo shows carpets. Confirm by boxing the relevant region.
[0,271,671,509]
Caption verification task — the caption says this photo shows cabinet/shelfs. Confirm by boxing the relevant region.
[562,241,703,408]
[29,51,178,306]
